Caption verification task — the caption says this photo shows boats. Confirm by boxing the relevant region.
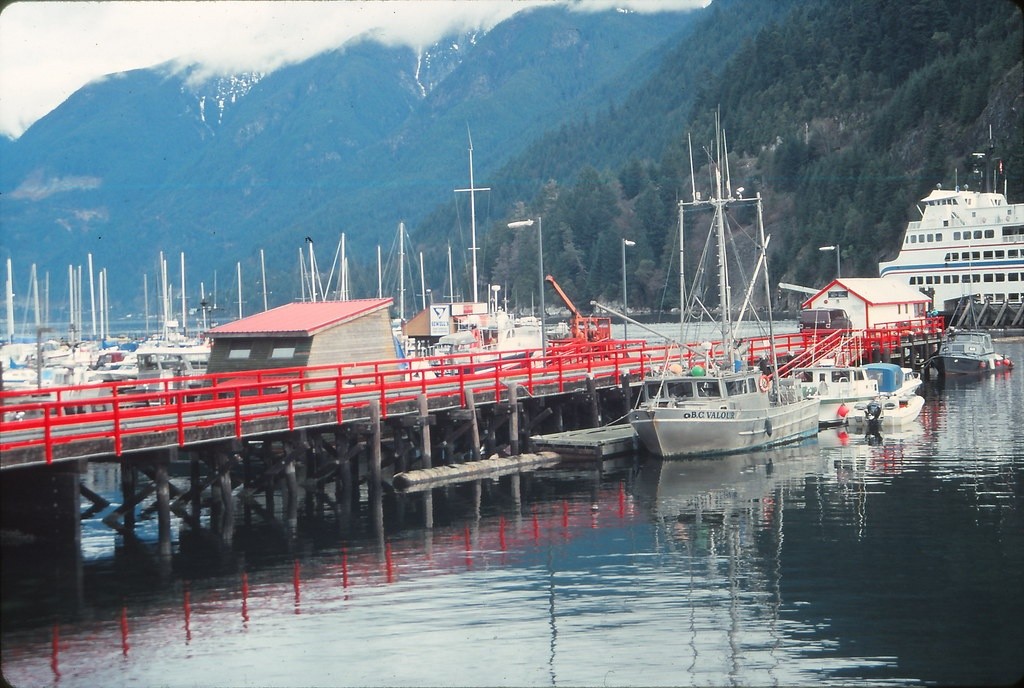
[625,99,820,458]
[862,364,923,398]
[879,121,1024,332]
[528,424,641,462]
[788,364,882,425]
[921,280,1012,380]
[847,398,927,431]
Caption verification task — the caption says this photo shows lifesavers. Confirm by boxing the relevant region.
[759,374,771,392]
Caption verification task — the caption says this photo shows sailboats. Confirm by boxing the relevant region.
[1,155,546,418]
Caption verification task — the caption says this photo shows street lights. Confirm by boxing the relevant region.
[506,217,548,379]
[621,239,637,359]
[820,246,840,280]
